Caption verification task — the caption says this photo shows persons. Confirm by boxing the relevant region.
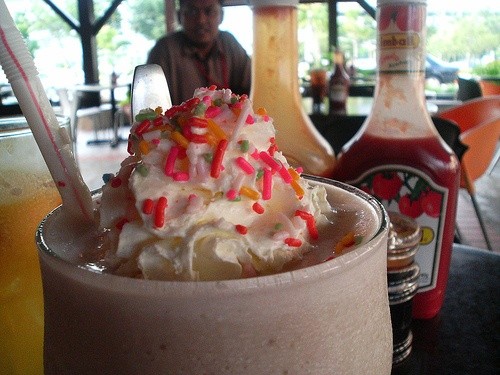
[147,0,253,107]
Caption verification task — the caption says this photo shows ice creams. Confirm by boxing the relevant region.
[95,84,332,282]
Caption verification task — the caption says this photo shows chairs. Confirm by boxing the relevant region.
[434,94,500,250]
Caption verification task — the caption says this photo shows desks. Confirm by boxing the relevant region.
[304,94,463,245]
[77,83,129,147]
[389,243,500,375]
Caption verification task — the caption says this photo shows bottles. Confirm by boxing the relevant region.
[386,214,420,363]
[112,72,116,84]
[328,53,349,108]
[323,0,461,318]
[248,0,334,180]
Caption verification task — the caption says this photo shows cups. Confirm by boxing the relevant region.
[0,114,72,375]
[309,67,328,103]
[33,170,395,375]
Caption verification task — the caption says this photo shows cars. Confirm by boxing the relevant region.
[345,48,459,89]
